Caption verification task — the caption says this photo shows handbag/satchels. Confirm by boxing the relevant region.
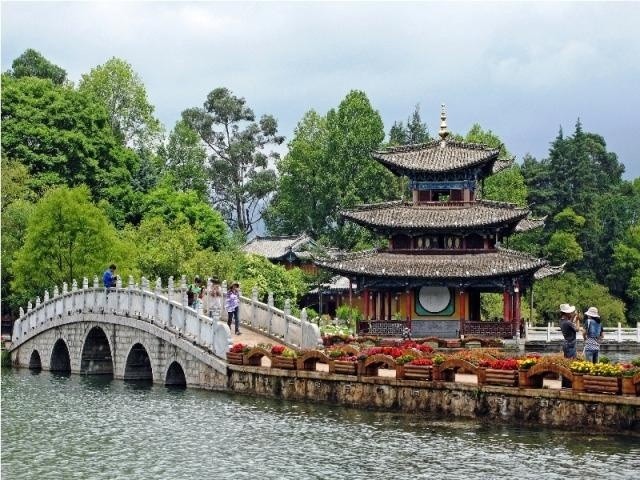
[584,337,601,352]
[188,292,194,306]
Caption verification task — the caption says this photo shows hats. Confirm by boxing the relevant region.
[559,302,576,315]
[583,307,600,318]
[229,281,240,290]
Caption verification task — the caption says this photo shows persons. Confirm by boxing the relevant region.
[558,303,579,359]
[186,277,206,311]
[582,306,604,364]
[225,281,241,335]
[103,264,116,287]
[208,274,224,322]
